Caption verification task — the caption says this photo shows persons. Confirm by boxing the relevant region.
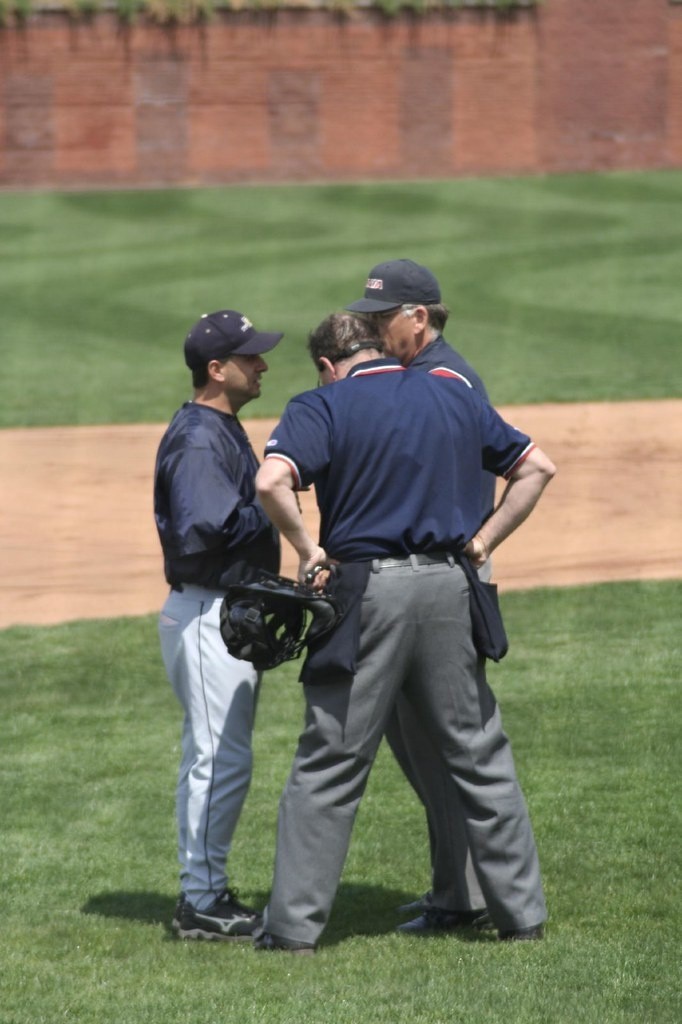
[152,308,302,942]
[254,308,560,957]
[344,256,494,938]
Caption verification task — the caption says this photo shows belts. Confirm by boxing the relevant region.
[369,551,452,570]
[170,583,183,594]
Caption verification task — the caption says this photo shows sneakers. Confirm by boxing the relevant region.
[171,890,263,942]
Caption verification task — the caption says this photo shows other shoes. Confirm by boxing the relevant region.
[398,905,495,935]
[398,889,435,912]
[254,929,313,957]
[464,917,546,941]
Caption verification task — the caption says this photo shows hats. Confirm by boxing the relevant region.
[343,258,441,313]
[184,312,284,371]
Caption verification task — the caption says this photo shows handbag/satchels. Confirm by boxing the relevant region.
[460,554,509,664]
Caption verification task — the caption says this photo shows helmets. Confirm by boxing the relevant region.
[219,568,343,671]
